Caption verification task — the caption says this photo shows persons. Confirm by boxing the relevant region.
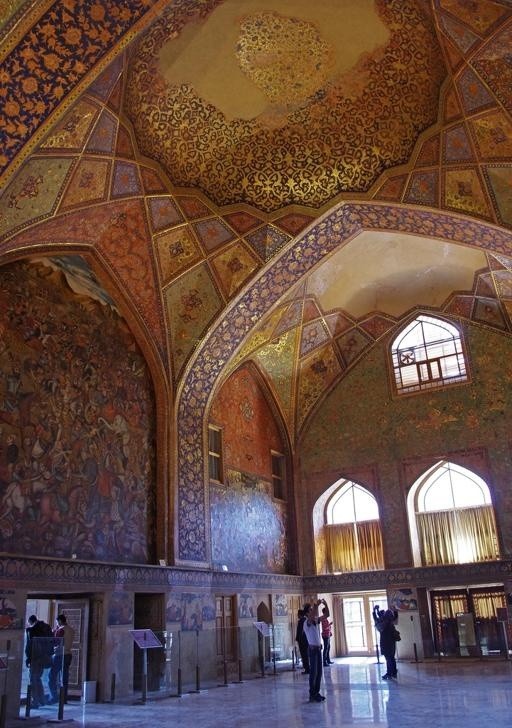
[25,613,76,709]
[294,599,333,702]
[371,603,403,680]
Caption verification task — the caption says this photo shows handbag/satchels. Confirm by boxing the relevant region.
[395,630,400,641]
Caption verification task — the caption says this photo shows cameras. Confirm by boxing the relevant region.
[375,606,379,608]
[320,600,324,603]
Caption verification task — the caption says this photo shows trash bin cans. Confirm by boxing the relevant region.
[84,681,97,703]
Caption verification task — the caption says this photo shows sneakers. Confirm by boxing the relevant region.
[323,661,334,667]
[300,669,311,675]
[381,671,397,681]
[309,694,325,703]
